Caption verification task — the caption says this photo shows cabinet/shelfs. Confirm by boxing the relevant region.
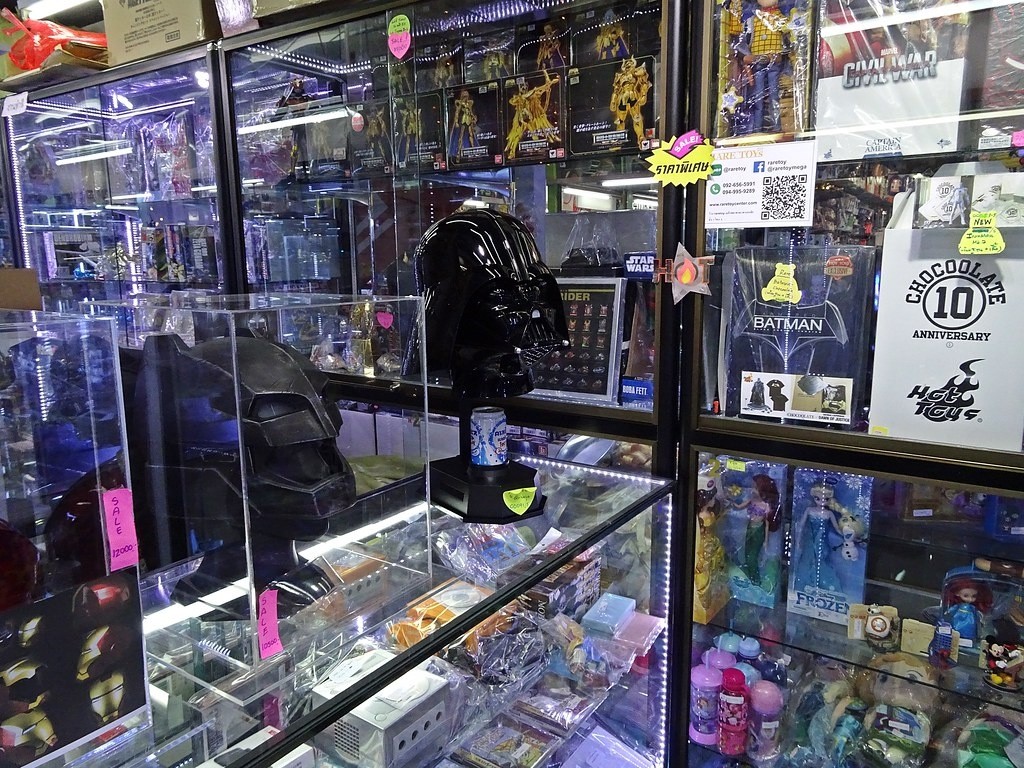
[677,443,1024,765]
[1,451,675,768]
[691,0,1024,490]
[320,398,667,767]
[0,38,240,373]
[218,0,675,440]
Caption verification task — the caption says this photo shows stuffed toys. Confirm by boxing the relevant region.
[857,633,1023,768]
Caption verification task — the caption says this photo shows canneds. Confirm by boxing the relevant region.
[470,406,508,469]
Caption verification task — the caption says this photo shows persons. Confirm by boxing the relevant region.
[727,474,865,606]
[730,0,794,133]
[944,580,994,639]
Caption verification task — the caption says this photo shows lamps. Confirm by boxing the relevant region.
[54,139,135,168]
[234,110,349,138]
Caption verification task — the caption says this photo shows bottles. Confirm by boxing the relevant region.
[689,629,786,762]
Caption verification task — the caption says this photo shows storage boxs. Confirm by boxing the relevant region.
[256,1,336,29]
[101,0,222,65]
